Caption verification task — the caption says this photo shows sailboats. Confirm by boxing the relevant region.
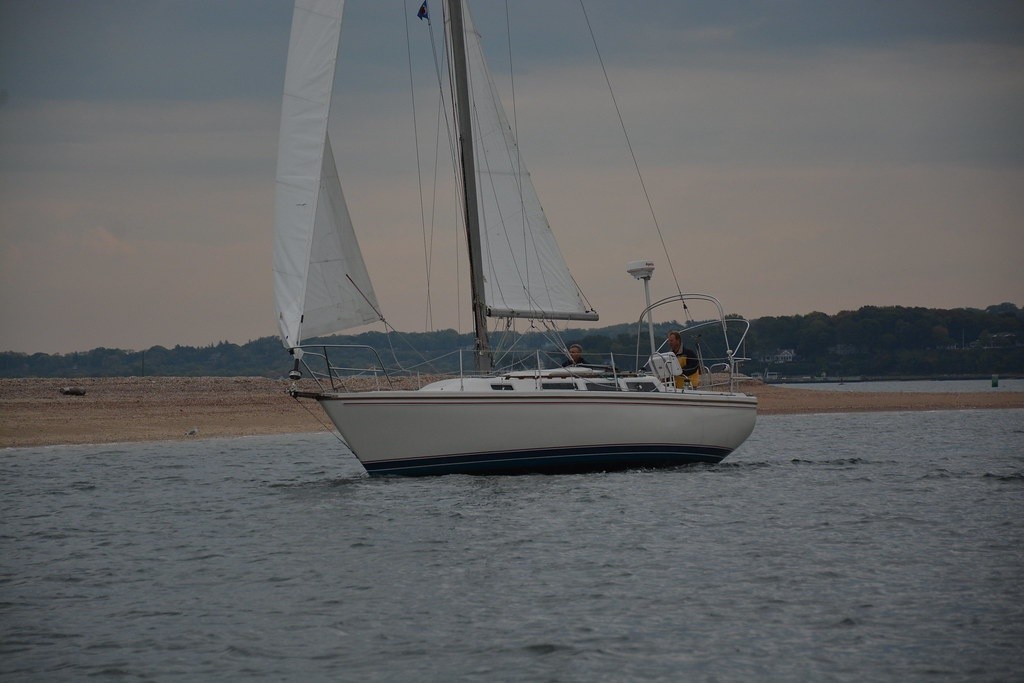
[272,0,757,475]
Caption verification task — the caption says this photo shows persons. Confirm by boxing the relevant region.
[561,344,593,369]
[667,331,701,391]
